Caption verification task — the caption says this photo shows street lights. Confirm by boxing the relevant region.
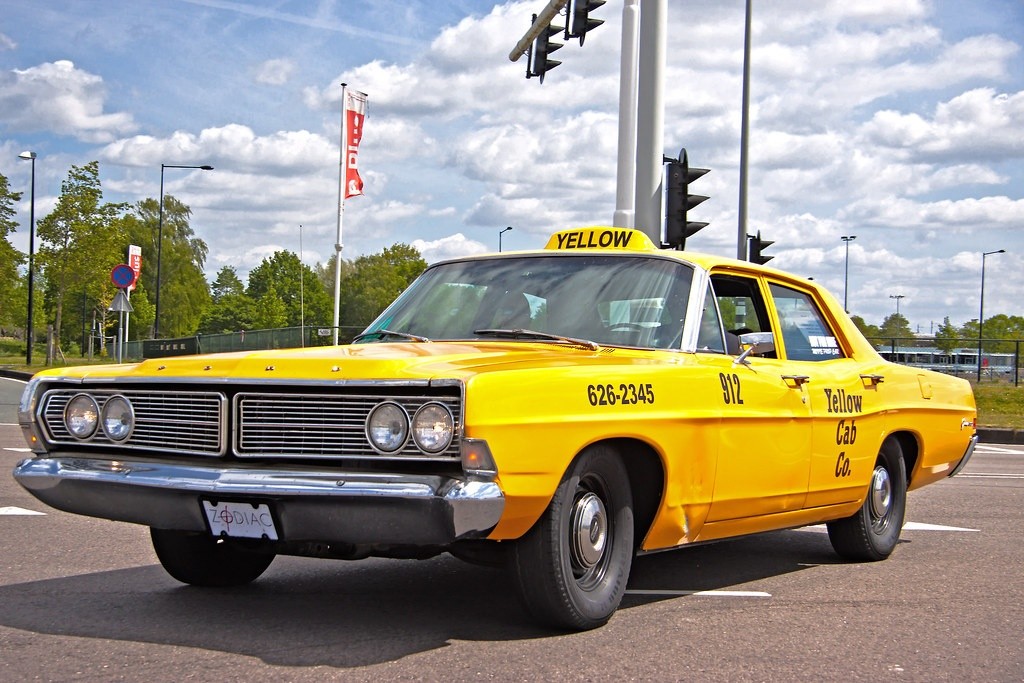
[153,164,215,336]
[18,151,37,364]
[497,226,514,252]
[839,236,857,310]
[890,294,904,364]
[977,249,1006,384]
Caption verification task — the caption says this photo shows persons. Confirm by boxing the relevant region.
[495,290,540,332]
[654,280,739,354]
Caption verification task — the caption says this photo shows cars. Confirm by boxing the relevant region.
[9,227,979,634]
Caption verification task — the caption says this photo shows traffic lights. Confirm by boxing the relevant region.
[663,149,713,250]
[529,24,563,81]
[570,0,604,48]
[745,232,778,263]
